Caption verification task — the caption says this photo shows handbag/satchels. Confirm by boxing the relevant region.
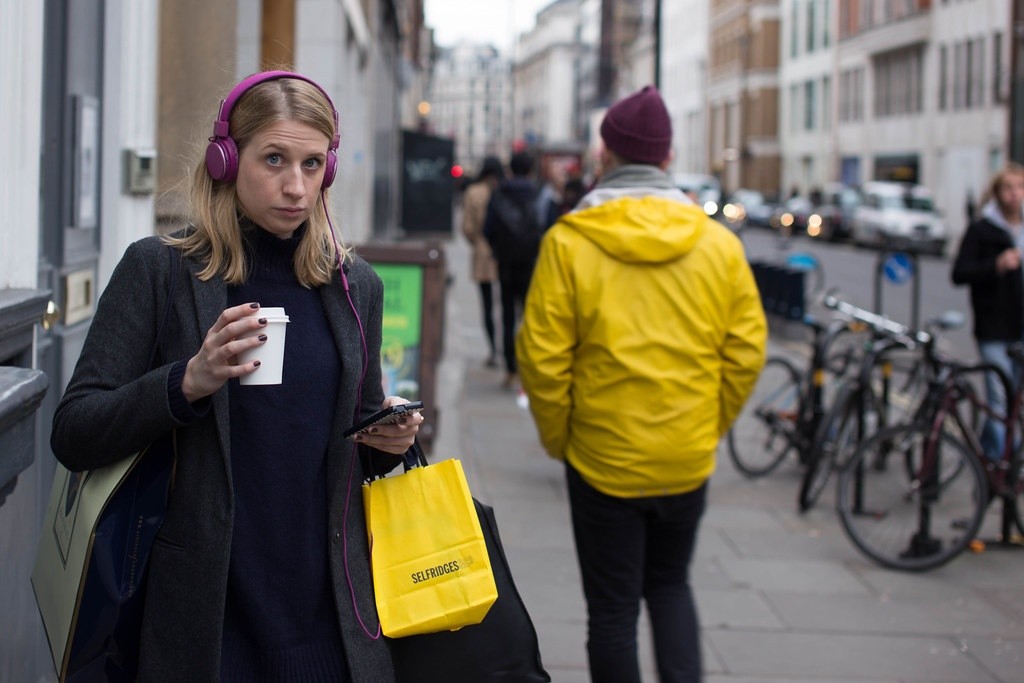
[361,447,498,638]
[388,436,552,683]
[30,437,171,683]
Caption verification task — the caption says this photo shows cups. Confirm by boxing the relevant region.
[237,308,290,386]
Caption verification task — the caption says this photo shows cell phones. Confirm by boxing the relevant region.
[342,401,425,440]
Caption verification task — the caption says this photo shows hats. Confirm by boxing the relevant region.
[600,87,672,162]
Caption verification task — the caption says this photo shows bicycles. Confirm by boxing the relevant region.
[728,286,1024,573]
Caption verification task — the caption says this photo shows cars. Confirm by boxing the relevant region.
[851,181,947,258]
[675,175,849,243]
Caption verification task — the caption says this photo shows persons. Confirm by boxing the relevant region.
[950,162,1024,506]
[461,151,604,408]
[52,70,422,683]
[518,85,767,682]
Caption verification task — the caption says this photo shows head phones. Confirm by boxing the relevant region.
[206,69,341,191]
